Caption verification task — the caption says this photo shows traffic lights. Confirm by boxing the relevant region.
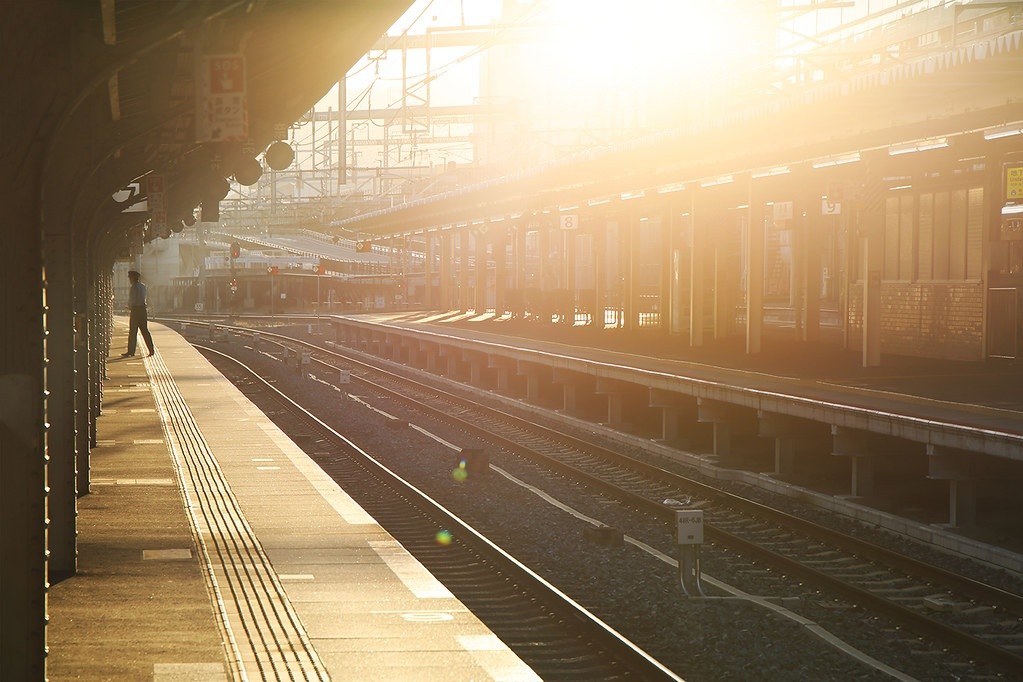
[230,242,241,260]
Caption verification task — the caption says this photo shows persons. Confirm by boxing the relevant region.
[122,271,154,356]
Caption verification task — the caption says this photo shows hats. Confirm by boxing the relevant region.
[128,271,140,277]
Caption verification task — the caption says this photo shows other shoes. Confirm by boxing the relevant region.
[121,352,134,357]
[148,352,154,357]
